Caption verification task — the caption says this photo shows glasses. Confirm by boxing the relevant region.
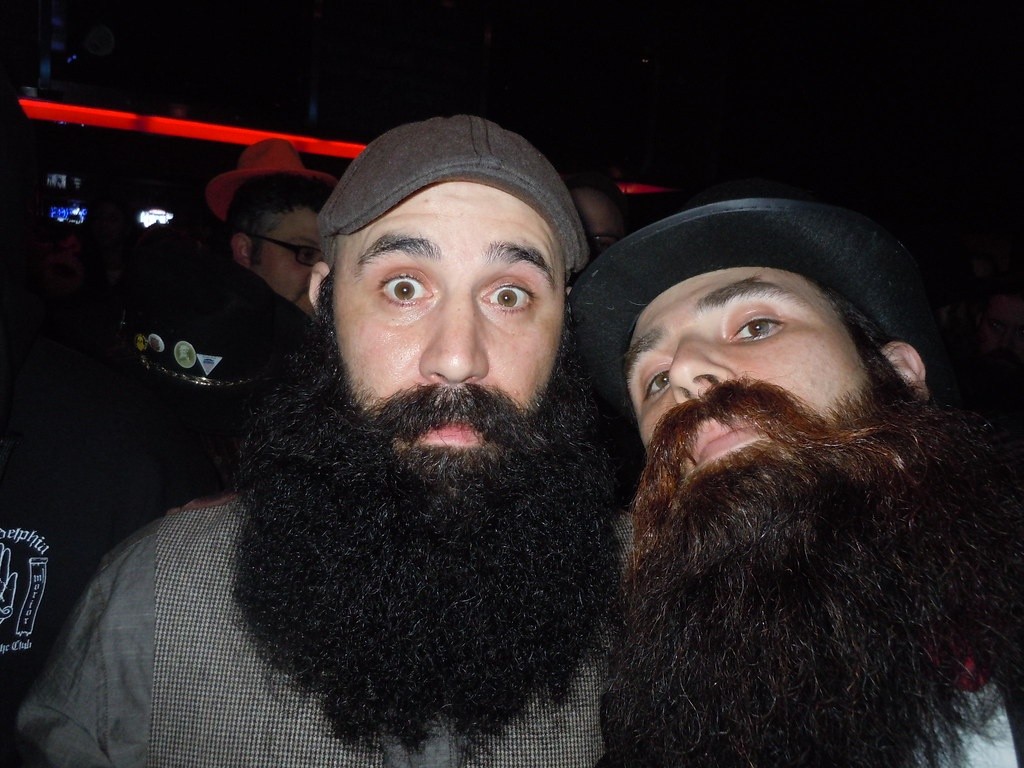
[583,233,619,255]
[239,228,320,265]
[982,315,1024,343]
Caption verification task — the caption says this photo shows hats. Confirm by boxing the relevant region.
[316,116,592,287]
[206,138,340,222]
[571,195,950,474]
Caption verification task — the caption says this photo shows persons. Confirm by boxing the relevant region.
[937,272,1024,410]
[12,116,637,768]
[562,172,627,260]
[0,137,340,768]
[569,198,1024,768]
